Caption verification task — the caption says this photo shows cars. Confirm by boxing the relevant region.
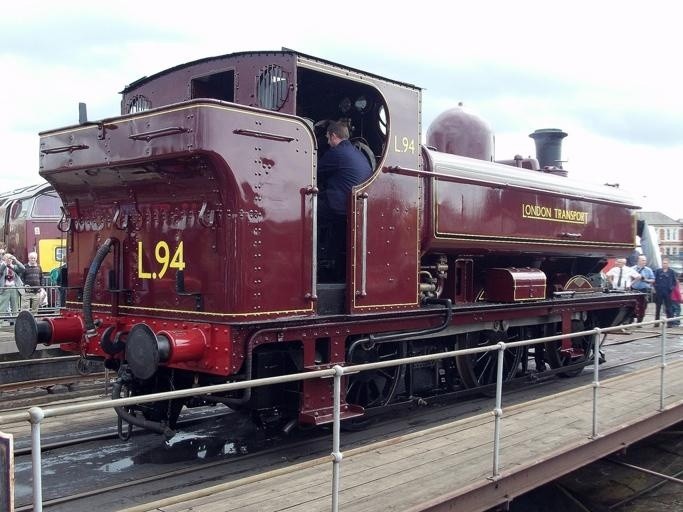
[667,259,683,282]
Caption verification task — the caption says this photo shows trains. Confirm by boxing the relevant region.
[0,180,67,307]
[12,44,650,457]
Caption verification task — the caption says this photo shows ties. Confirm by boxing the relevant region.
[618,269,622,286]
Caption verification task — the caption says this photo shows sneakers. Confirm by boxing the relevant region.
[654,322,680,328]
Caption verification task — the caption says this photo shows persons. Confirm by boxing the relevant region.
[669,270,683,326]
[650,257,677,328]
[604,258,641,333]
[628,255,656,328]
[0,241,67,329]
[315,121,373,282]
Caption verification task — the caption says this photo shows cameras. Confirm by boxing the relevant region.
[641,276,645,281]
[8,255,12,259]
